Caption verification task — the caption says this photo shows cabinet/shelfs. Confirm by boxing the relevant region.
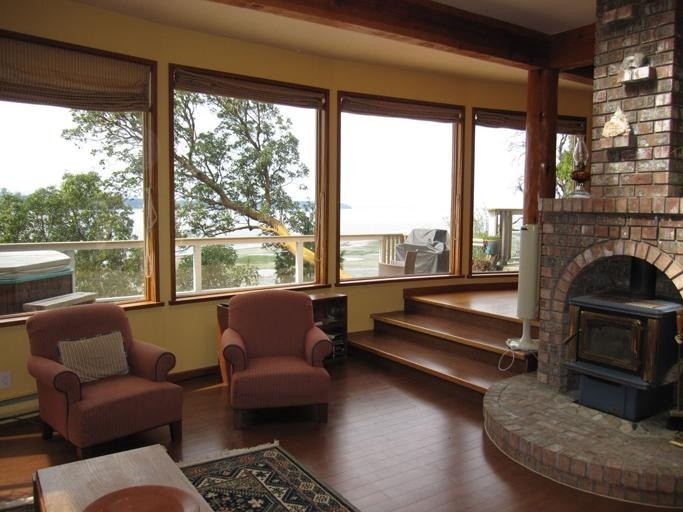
[216,290,348,366]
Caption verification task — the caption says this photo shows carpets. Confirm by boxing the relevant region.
[0,440,360,511]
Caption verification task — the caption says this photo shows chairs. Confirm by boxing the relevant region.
[219,290,333,430]
[379,251,416,274]
[26,303,183,461]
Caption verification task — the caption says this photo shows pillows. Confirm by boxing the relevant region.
[56,330,130,386]
